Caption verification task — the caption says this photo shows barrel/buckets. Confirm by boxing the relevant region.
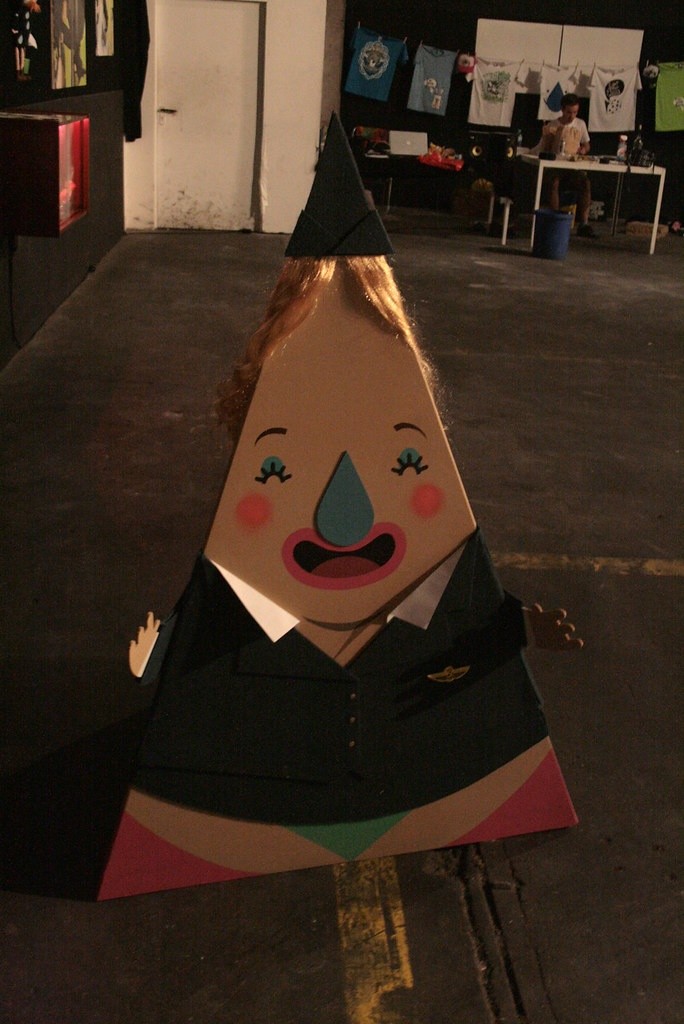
[533,209,573,260]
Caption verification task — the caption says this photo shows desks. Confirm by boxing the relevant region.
[501,154,665,254]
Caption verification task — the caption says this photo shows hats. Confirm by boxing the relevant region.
[369,128,390,149]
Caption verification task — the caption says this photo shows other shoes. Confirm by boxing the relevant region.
[577,224,601,239]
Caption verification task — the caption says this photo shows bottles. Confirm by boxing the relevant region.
[633,124,643,151]
[516,129,523,146]
[617,135,628,161]
[559,137,566,154]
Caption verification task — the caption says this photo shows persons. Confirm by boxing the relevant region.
[539,94,597,237]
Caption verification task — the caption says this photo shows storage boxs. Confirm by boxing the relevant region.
[626,221,668,239]
